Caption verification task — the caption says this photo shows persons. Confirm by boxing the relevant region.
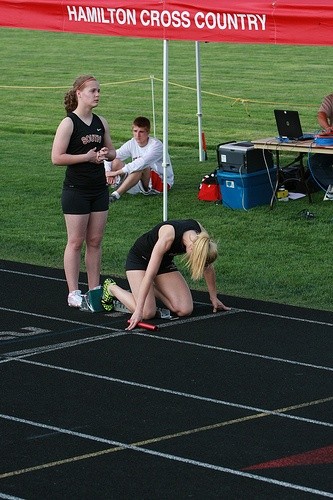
[309,95,333,199]
[51,74,116,307]
[102,116,174,203]
[101,220,231,330]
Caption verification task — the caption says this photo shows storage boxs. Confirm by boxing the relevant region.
[217,170,276,210]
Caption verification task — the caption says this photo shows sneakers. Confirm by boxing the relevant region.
[68,289,83,308]
[102,278,116,312]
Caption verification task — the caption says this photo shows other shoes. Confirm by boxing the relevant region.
[108,190,120,203]
[323,184,333,201]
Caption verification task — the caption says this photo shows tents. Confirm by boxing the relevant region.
[0,0,333,220]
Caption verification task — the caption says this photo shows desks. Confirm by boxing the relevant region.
[251,137,333,210]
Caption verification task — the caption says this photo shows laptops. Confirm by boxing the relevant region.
[273,110,315,140]
